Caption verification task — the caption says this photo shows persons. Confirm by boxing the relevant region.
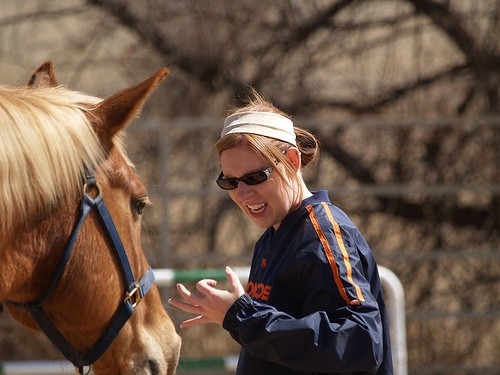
[167,99,394,375]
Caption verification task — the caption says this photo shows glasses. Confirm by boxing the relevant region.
[216,144,294,191]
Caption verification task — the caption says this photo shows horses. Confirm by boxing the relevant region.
[0,60,183,374]
